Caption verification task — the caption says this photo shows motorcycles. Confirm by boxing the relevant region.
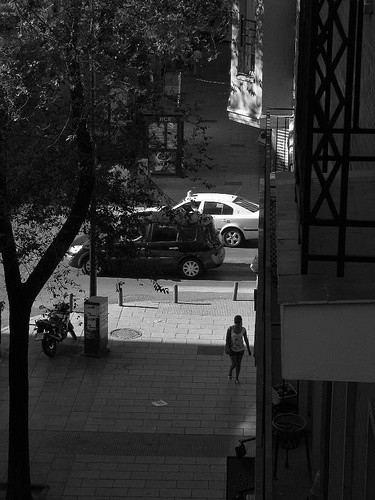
[34,301,77,358]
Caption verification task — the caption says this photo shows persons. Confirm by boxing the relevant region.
[225,314,253,384]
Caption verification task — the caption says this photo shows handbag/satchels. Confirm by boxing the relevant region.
[225,326,232,355]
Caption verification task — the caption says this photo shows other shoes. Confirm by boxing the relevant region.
[228,376,232,381]
[235,380,241,384]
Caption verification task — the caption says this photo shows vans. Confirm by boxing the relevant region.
[65,211,225,279]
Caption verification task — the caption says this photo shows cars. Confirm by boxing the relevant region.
[257,130,266,156]
[113,190,259,247]
